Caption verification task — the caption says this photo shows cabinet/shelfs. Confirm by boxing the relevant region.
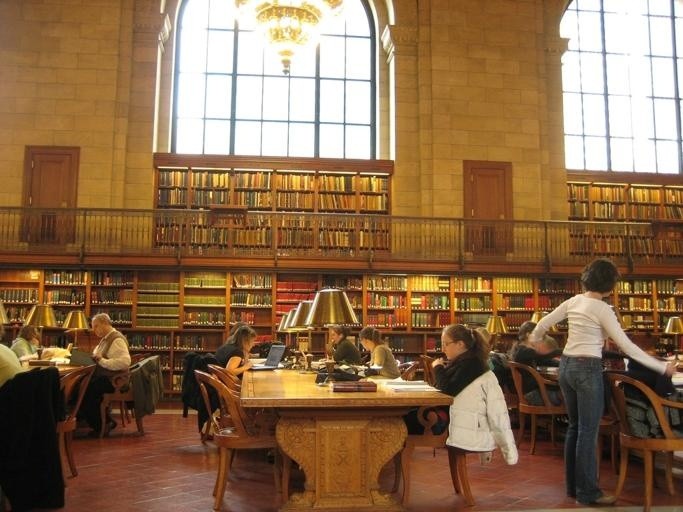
[565,168,682,264]
[0,267,683,404]
[152,151,393,256]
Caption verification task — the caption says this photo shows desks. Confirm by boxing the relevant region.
[235,353,456,511]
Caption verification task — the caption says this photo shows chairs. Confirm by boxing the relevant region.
[0,354,160,495]
[184,353,292,510]
[601,372,683,511]
[501,356,618,481]
[386,355,478,509]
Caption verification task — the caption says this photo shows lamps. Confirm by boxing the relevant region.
[231,1,347,77]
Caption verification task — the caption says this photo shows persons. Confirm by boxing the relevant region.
[511,321,562,408]
[529,258,676,507]
[402,324,490,436]
[73,313,131,440]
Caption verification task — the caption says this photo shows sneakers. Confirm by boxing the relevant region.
[576,493,616,506]
[89,419,116,438]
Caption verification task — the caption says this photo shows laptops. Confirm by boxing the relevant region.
[250,345,285,371]
